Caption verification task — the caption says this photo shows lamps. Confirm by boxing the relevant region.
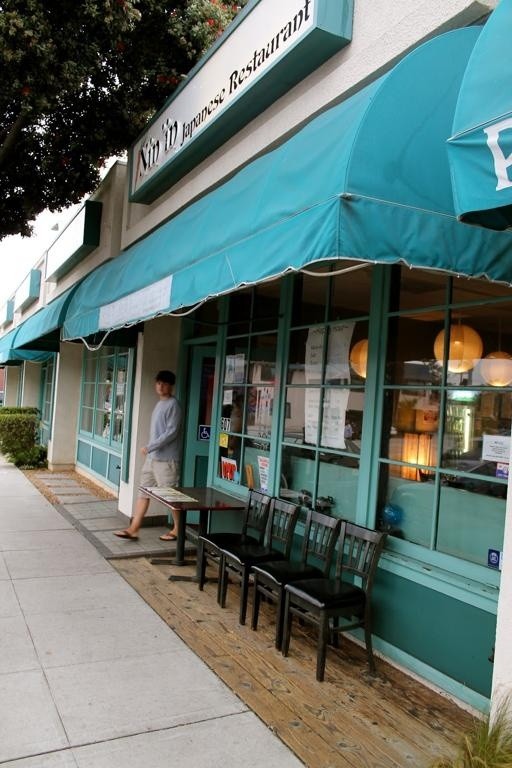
[349,339,386,379]
[482,302,512,386]
[434,275,481,374]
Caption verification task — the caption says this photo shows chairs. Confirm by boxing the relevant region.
[199,489,386,683]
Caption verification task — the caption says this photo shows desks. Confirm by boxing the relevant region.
[140,487,257,561]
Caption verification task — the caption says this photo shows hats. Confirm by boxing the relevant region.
[157,371,175,385]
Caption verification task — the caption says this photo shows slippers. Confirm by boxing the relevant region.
[160,533,187,541]
[114,531,137,540]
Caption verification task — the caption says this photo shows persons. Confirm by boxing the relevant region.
[344,413,353,441]
[227,396,241,454]
[113,370,184,540]
